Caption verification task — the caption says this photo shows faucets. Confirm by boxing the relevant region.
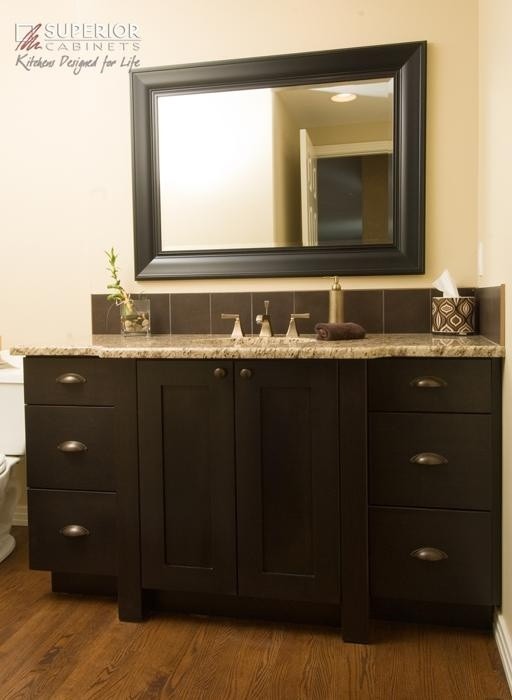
[255,300,272,336]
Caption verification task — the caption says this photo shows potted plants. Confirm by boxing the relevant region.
[102,247,151,337]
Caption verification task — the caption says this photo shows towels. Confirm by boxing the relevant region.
[315,322,368,341]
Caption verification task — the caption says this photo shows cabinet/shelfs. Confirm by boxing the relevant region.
[22,355,137,590]
[366,356,503,631]
[137,354,342,605]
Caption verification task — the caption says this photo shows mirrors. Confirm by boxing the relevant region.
[127,38,428,281]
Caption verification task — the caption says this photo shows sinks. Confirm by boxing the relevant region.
[188,334,320,347]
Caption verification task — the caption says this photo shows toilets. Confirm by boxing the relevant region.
[0,368,29,564]
